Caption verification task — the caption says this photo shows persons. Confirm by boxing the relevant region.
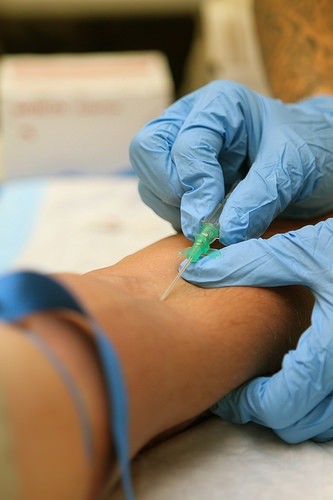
[131,79,332,444]
[0,215,325,500]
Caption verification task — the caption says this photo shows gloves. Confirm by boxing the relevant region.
[177,216,332,444]
[127,77,333,246]
